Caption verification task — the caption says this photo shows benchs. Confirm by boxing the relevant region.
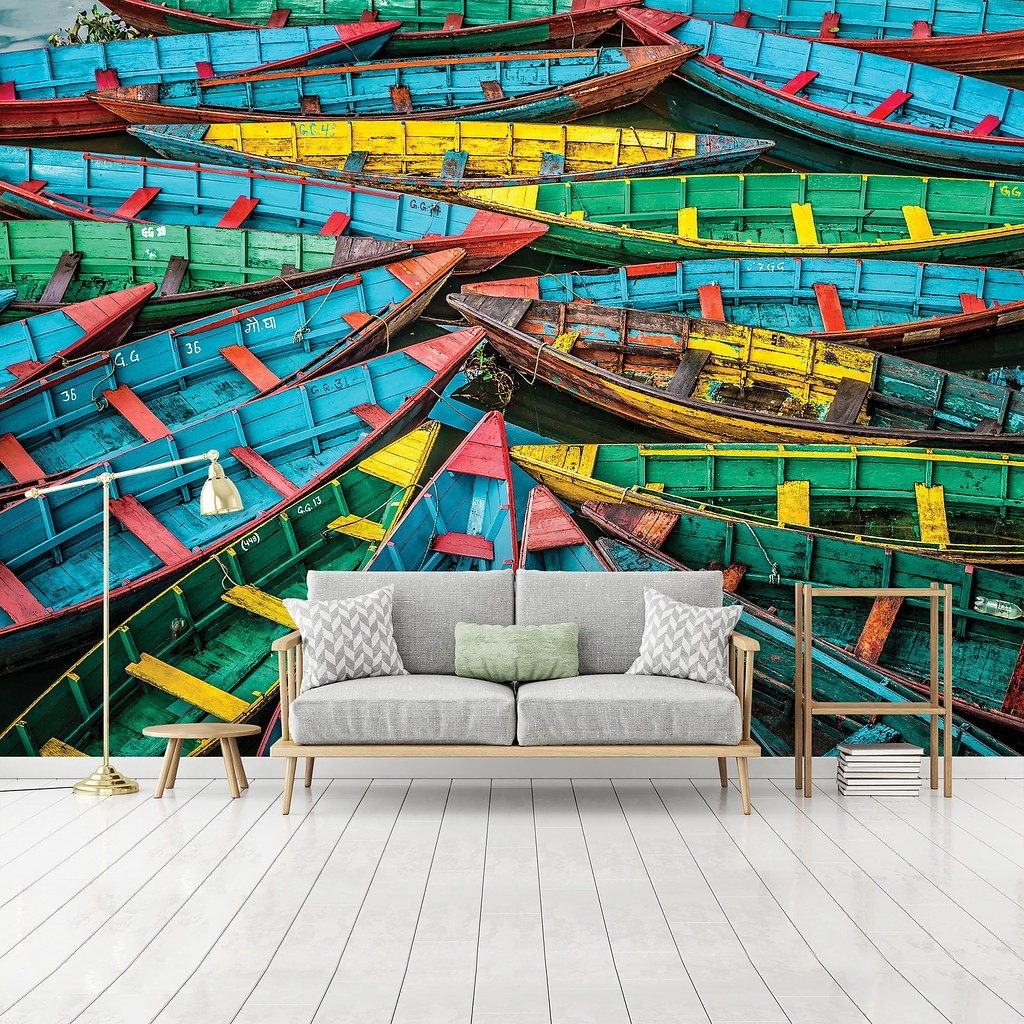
[266,568,762,815]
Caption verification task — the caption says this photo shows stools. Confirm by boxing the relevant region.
[141,724,263,797]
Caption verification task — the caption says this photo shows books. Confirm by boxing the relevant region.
[836,743,925,797]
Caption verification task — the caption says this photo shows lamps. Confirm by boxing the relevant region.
[24,451,246,799]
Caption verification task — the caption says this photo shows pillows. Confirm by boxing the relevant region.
[307,570,514,679]
[515,569,724,674]
[624,584,744,692]
[282,583,410,695]
[453,621,578,683]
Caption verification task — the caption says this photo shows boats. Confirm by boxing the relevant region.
[2,0,1023,756]
